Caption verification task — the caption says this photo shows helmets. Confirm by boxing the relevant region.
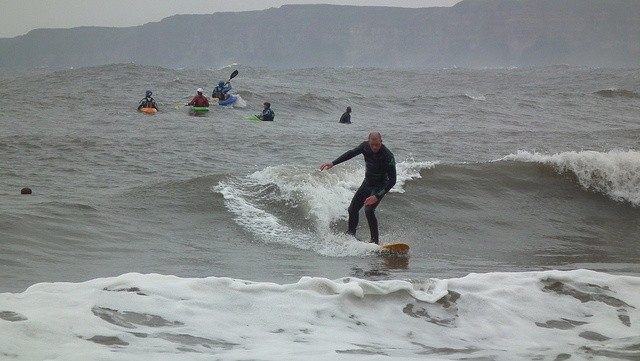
[197,88,204,93]
[218,80,225,86]
[145,90,153,97]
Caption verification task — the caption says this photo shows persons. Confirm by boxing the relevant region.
[137,90,160,113]
[185,88,209,108]
[212,81,232,101]
[339,107,352,124]
[255,102,275,122]
[319,133,396,245]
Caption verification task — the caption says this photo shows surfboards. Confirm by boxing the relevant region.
[378,242,409,256]
